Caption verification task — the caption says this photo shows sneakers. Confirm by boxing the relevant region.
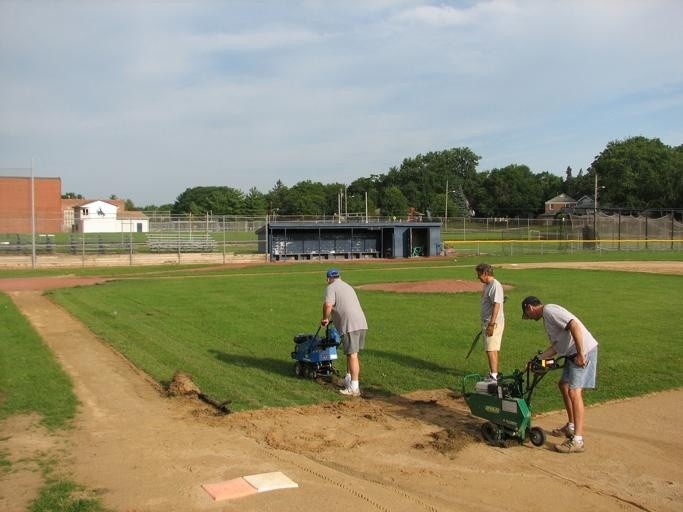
[336,379,361,397]
[554,425,584,453]
[475,379,497,390]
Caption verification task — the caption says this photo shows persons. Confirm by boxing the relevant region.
[475,263,508,377]
[520,294,599,453]
[321,268,368,397]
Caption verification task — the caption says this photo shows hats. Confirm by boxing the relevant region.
[521,296,539,320]
[327,269,340,277]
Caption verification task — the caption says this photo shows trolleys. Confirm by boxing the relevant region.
[412,244,425,256]
[462,351,567,447]
[291,321,342,380]
